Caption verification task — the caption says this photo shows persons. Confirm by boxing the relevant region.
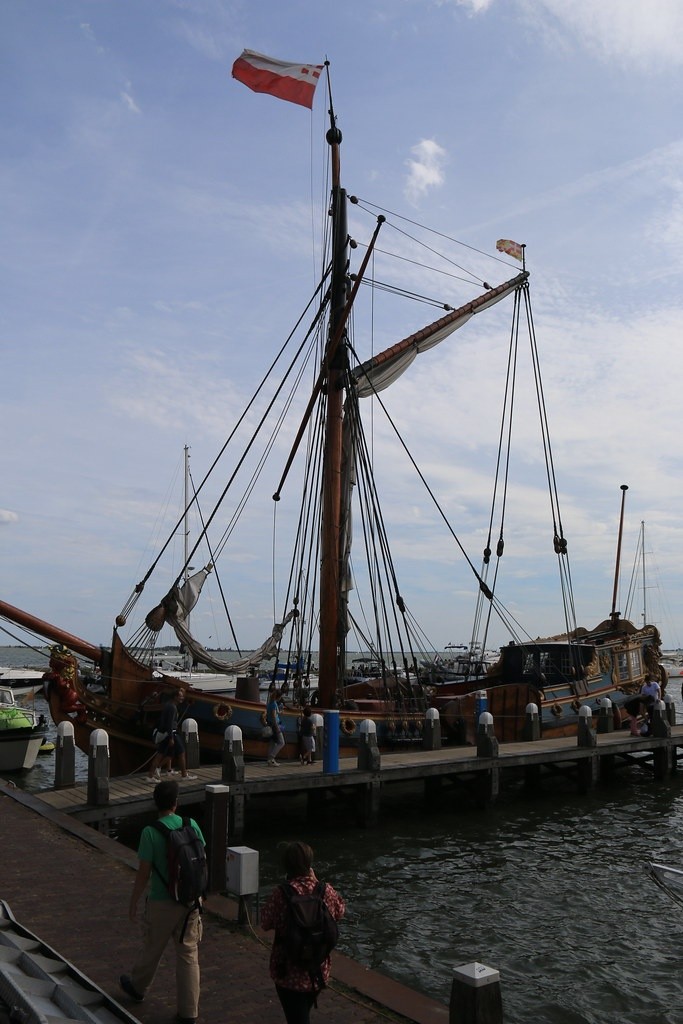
[625,695,654,738]
[299,707,315,766]
[265,689,285,767]
[641,676,661,736]
[146,687,198,783]
[261,840,345,1024]
[119,780,205,1024]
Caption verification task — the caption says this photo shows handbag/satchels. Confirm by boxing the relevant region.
[254,720,273,742]
[638,716,654,737]
[154,731,170,745]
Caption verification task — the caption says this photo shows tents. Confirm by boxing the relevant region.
[352,658,379,664]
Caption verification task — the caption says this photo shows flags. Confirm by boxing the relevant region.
[496,239,523,262]
[231,48,324,110]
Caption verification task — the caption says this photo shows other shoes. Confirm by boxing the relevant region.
[154,767,162,779]
[118,974,145,1003]
[172,1013,196,1024]
[181,773,198,782]
[146,776,162,784]
[166,769,179,776]
[631,729,641,737]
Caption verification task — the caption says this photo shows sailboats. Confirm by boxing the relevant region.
[1,50,683,811]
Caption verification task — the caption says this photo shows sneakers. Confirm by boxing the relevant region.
[307,759,318,766]
[267,758,282,767]
[299,753,306,767]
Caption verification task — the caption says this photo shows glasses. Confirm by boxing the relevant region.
[646,681,651,684]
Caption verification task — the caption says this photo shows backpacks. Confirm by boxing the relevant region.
[145,816,210,905]
[278,879,340,1009]
